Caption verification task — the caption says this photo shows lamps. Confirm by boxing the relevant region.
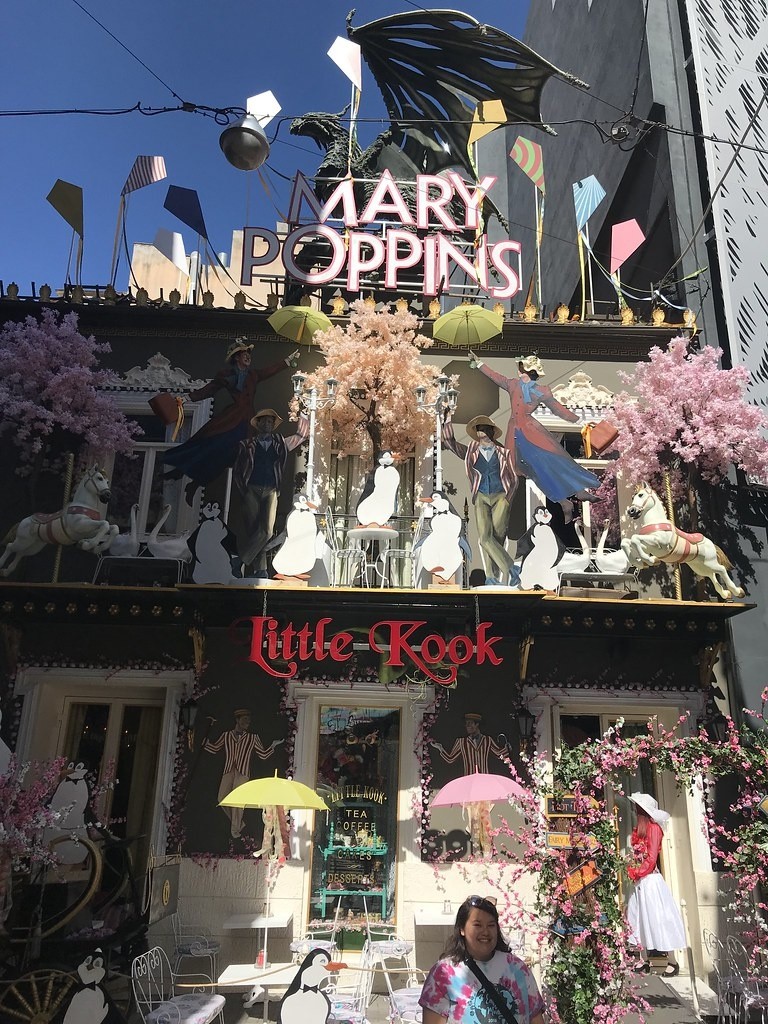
[219,112,272,171]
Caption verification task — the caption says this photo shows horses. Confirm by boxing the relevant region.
[0,462,120,578]
[621,480,745,600]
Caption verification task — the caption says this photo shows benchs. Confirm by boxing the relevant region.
[92,532,191,586]
[556,547,640,599]
[377,944,426,1024]
[317,939,377,1024]
[132,946,226,1024]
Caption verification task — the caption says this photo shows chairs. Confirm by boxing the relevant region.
[381,516,424,589]
[703,928,768,1024]
[360,896,414,1008]
[171,913,221,995]
[289,896,343,975]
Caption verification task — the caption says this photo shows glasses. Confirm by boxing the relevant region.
[467,895,497,908]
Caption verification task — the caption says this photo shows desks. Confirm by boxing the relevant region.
[325,505,369,589]
[223,914,292,956]
[217,963,299,1024]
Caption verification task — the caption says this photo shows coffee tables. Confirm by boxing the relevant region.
[346,528,399,589]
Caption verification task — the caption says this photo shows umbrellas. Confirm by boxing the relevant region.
[433,303,505,369]
[216,768,330,858]
[428,765,534,845]
[266,305,335,365]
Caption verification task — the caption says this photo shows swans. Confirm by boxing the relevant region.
[110,503,140,557]
[556,520,591,572]
[147,504,192,560]
[595,519,630,573]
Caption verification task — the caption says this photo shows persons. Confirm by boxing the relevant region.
[627,791,687,977]
[418,895,548,1024]
